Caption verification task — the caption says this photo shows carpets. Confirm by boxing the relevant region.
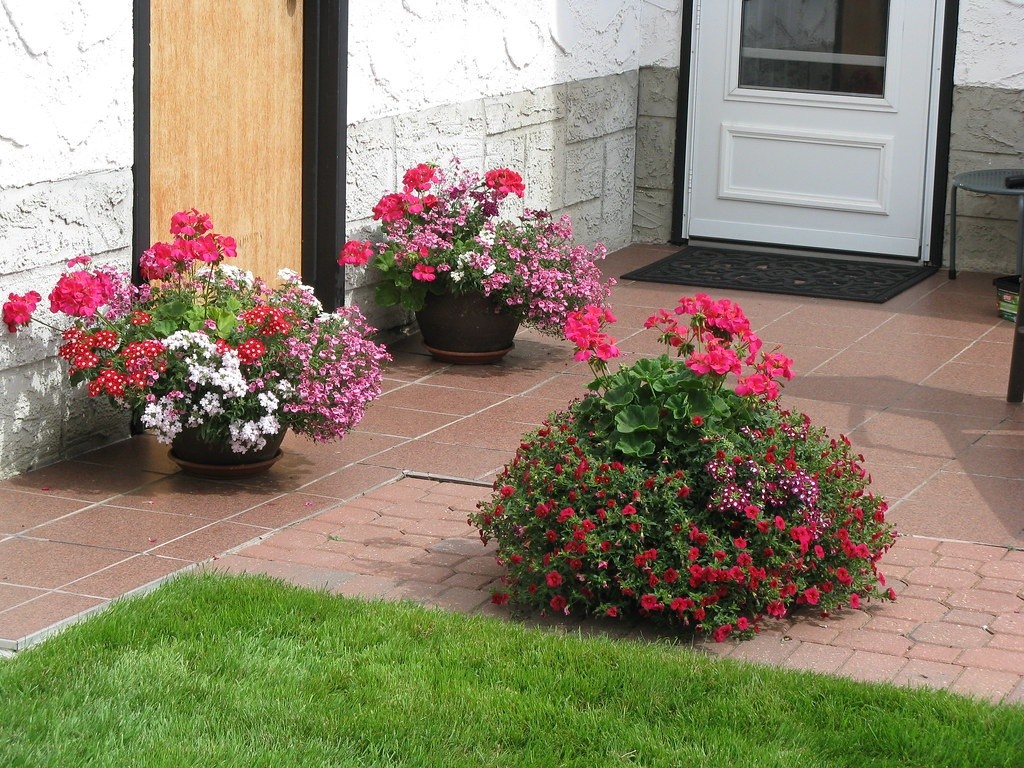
[620,246,940,304]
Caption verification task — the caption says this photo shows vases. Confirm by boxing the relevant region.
[167,423,289,481]
[413,296,520,366]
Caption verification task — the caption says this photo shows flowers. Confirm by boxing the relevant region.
[467,292,900,642]
[2,208,393,456]
[336,156,618,339]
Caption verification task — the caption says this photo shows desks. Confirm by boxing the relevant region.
[948,169,1024,279]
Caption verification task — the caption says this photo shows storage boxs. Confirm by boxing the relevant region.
[993,275,1021,322]
